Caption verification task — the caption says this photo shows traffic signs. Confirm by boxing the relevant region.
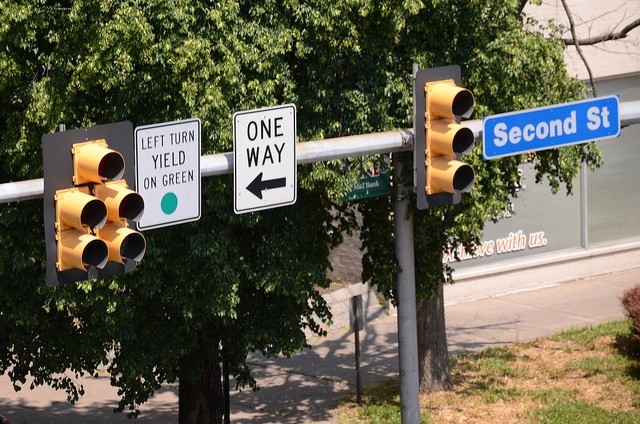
[134,117,201,231]
[482,94,621,160]
[233,103,298,216]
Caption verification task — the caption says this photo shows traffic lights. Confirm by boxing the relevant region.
[415,65,476,210]
[43,120,147,288]
